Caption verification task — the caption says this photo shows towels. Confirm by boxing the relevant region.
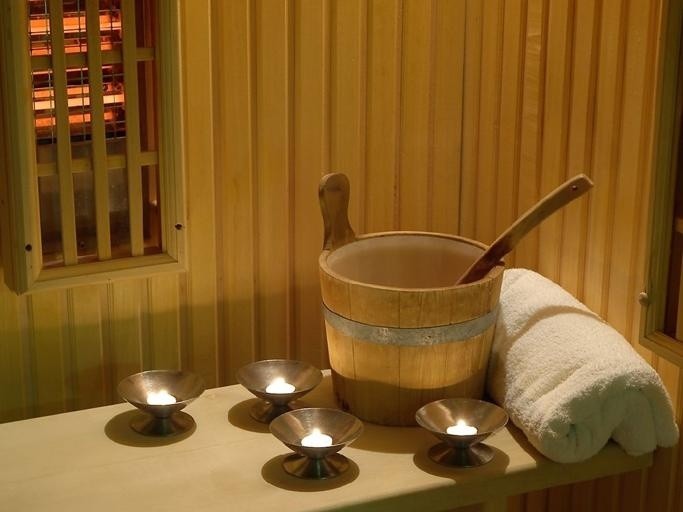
[484,267,683,467]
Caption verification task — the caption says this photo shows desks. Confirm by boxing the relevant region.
[1,368,654,511]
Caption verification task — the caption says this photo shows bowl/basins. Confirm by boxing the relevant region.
[233,355,325,426]
[264,405,364,478]
[414,395,511,469]
[111,360,208,440]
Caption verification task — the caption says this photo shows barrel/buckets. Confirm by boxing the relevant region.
[316,171,504,425]
